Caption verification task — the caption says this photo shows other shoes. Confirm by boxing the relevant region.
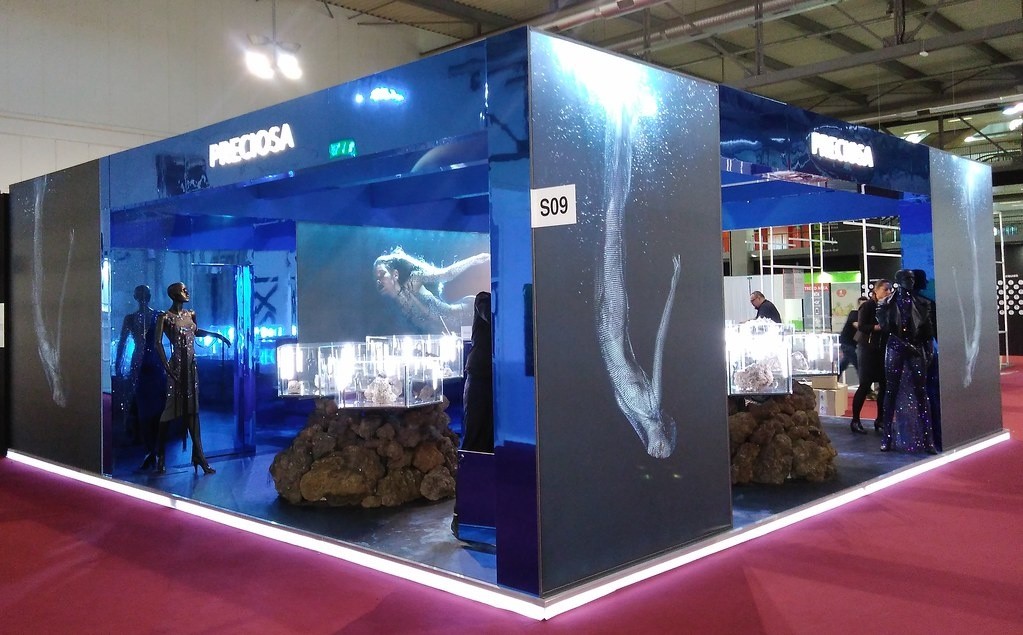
[874,419,885,431]
[850,420,867,434]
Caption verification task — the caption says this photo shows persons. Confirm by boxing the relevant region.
[912,268,941,343]
[738,291,784,324]
[876,268,941,455]
[113,285,188,477]
[849,279,896,434]
[593,102,685,460]
[460,292,495,453]
[837,295,878,401]
[369,249,492,334]
[152,281,231,477]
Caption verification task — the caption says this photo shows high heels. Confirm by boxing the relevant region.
[140,452,155,469]
[155,452,167,476]
[192,456,216,475]
